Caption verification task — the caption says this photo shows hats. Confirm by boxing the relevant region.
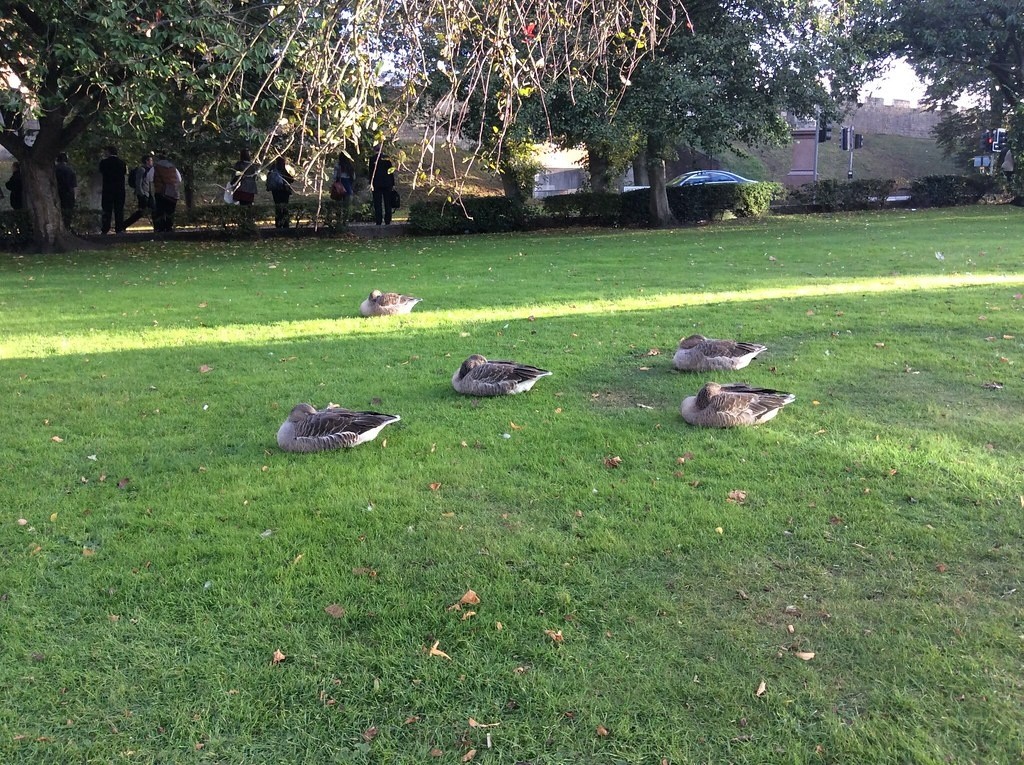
[56,152,68,163]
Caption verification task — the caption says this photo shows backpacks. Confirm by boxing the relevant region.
[128,166,145,188]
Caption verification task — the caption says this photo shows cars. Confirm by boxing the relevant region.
[666,168,756,187]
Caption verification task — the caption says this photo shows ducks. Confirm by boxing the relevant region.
[360,290,424,317]
[672,334,768,371]
[276,403,401,453]
[682,382,796,428]
[452,354,554,396]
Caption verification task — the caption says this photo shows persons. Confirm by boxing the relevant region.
[333,151,357,225]
[370,145,394,225]
[99,146,126,234]
[121,155,181,232]
[55,152,78,234]
[6,161,22,210]
[269,149,292,227]
[230,150,257,206]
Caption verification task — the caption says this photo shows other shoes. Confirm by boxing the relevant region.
[117,231,130,236]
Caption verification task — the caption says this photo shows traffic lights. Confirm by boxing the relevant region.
[819,123,832,143]
[991,128,1010,152]
[856,133,864,149]
[839,127,855,154]
[981,132,992,152]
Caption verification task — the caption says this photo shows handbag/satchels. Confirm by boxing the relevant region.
[267,168,293,196]
[331,181,347,200]
[240,178,257,194]
[224,182,234,203]
[390,189,401,213]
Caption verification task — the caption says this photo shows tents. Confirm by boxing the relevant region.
[520,143,588,199]
